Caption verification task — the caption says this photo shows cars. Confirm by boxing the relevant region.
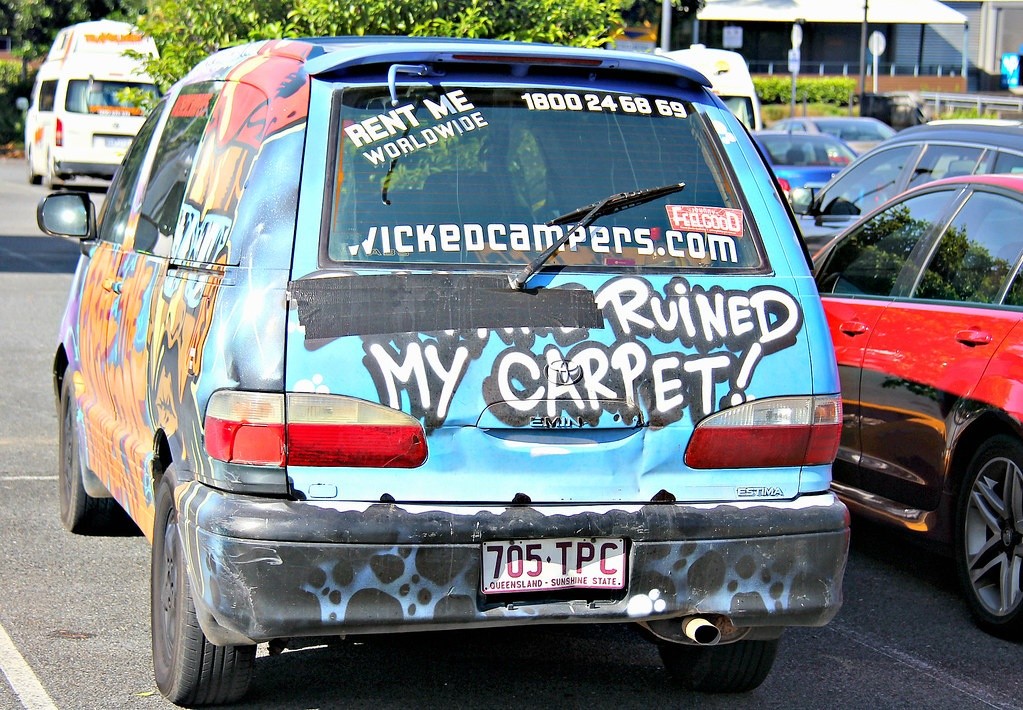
[39,39,852,704]
[744,113,1022,259]
[804,172,1023,642]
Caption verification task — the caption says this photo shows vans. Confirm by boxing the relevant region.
[655,44,762,131]
[15,17,162,187]
